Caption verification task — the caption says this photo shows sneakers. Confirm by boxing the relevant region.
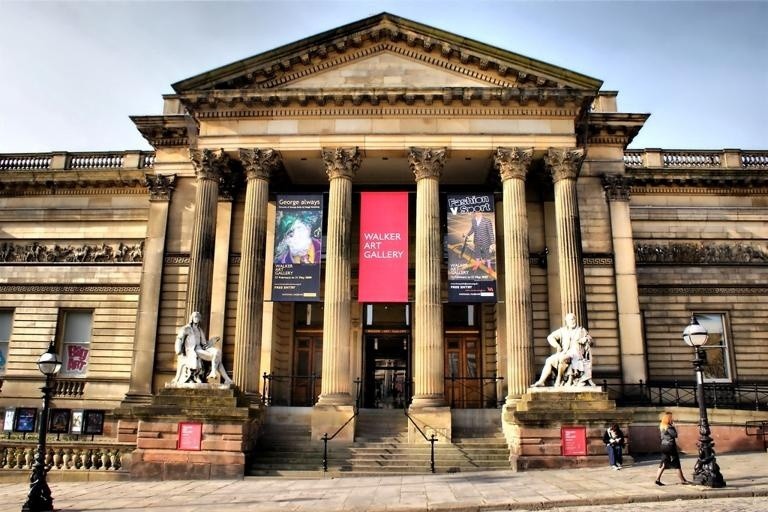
[653,480,667,486]
[609,463,623,471]
[681,481,693,486]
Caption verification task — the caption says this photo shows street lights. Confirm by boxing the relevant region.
[682,312,725,487]
[19,339,63,512]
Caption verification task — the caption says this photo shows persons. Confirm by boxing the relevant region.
[603,423,626,470]
[174,311,235,384]
[464,212,496,275]
[529,313,589,387]
[655,411,690,485]
